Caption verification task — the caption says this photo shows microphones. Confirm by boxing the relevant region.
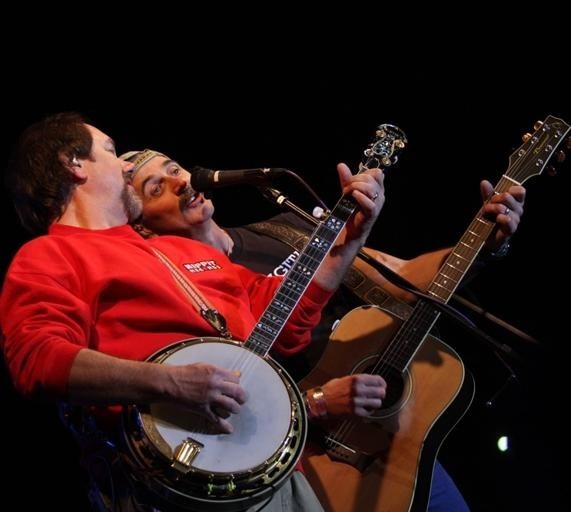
[190,168,286,191]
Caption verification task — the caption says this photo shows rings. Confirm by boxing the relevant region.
[373,191,379,200]
[504,207,510,214]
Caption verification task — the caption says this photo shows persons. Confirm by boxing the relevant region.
[117,149,528,510]
[1,110,385,512]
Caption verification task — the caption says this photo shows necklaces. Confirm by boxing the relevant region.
[222,232,232,258]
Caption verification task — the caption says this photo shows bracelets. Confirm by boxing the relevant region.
[314,385,327,418]
[301,391,313,419]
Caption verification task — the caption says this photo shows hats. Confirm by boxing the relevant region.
[117,149,171,184]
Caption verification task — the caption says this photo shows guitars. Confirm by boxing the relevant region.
[295,115,570,510]
